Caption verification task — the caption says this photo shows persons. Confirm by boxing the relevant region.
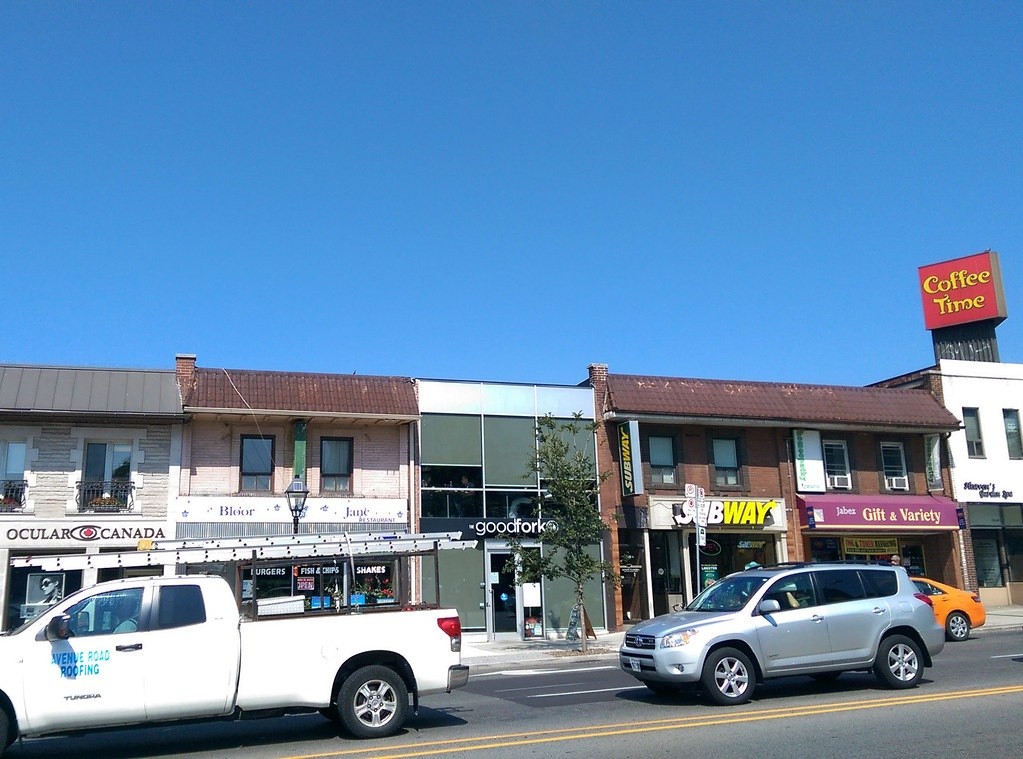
[706,578,715,586]
[891,555,900,566]
[36,575,62,605]
[110,596,138,634]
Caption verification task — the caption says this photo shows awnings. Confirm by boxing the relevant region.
[795,493,960,532]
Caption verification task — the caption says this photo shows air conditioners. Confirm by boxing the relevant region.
[830,475,849,488]
[887,477,906,489]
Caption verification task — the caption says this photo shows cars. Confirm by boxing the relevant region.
[256,587,308,599]
[911,577,987,640]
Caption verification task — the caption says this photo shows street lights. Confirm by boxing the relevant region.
[285,474,311,592]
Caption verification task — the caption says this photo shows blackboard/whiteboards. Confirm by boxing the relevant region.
[566,604,595,638]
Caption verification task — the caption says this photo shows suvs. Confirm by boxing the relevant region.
[618,556,946,705]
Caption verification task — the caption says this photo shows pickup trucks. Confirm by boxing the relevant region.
[0,572,469,758]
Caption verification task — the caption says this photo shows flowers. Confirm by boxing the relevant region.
[0,498,19,506]
[350,575,394,598]
[87,492,123,507]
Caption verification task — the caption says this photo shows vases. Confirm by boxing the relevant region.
[92,504,119,512]
[0,506,15,512]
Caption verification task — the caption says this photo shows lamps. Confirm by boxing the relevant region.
[284,475,310,534]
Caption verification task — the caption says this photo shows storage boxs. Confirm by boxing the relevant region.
[311,596,330,608]
[350,594,365,605]
[374,597,395,603]
